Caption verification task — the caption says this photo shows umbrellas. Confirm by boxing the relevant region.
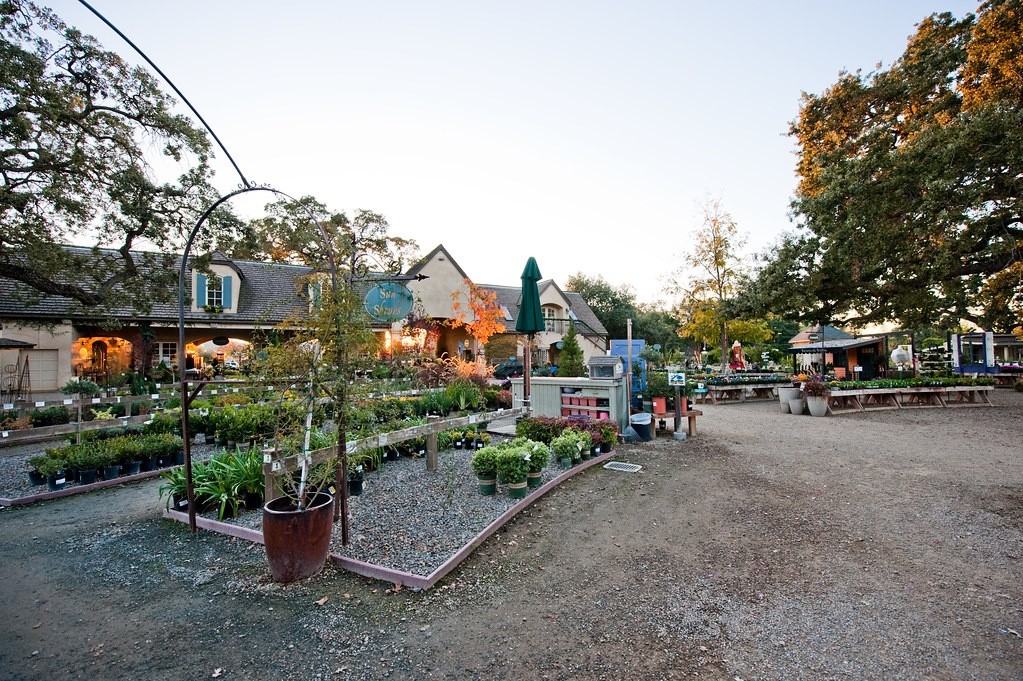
[516,256,545,402]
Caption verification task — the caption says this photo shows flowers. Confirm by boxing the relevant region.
[830,381,837,386]
[791,374,822,384]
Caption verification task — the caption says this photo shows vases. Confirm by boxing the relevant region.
[831,387,838,391]
[794,383,801,388]
[789,399,804,415]
[777,387,799,413]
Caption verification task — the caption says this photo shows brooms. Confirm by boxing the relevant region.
[617,373,643,443]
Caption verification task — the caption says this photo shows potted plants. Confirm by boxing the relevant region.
[803,382,830,417]
[669,379,693,413]
[27,274,622,582]
[646,372,670,414]
[838,375,993,387]
